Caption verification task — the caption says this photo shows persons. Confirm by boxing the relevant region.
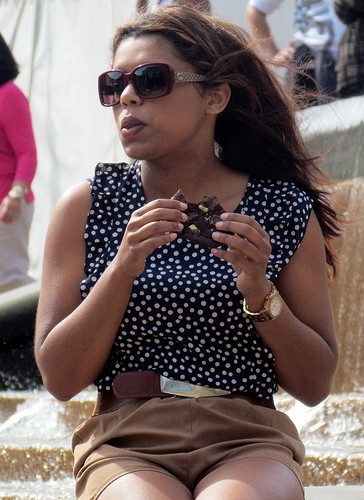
[247,1,346,109]
[333,0,364,100]
[136,1,212,17]
[31,0,341,500]
[0,33,38,295]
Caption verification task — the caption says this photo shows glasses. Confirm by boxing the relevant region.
[98,64,208,106]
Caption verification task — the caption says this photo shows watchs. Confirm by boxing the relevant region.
[8,186,24,199]
[243,280,283,322]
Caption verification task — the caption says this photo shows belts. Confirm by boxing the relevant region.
[109,372,245,398]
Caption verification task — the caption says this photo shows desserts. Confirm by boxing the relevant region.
[169,190,230,247]
